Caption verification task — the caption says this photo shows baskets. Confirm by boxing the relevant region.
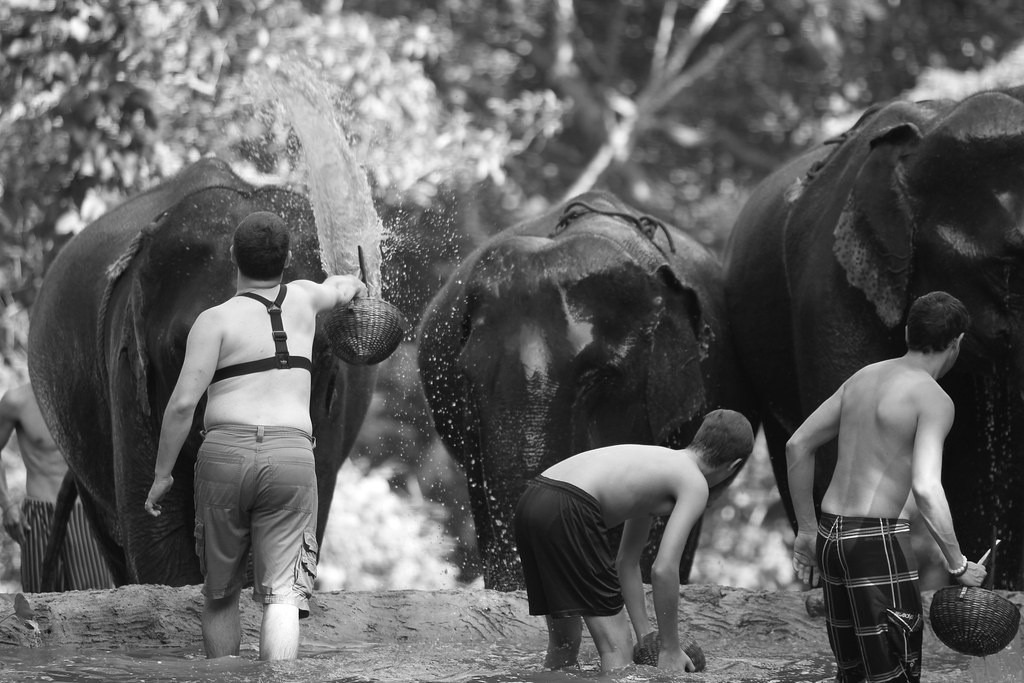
[927,538,1020,657]
[324,245,404,366]
[633,628,707,674]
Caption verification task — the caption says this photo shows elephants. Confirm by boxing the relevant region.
[25,87,1024,589]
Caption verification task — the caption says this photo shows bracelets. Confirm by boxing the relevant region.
[945,554,968,578]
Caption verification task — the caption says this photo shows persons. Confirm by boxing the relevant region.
[0,380,119,594]
[144,210,369,669]
[514,408,756,677]
[785,290,988,682]
